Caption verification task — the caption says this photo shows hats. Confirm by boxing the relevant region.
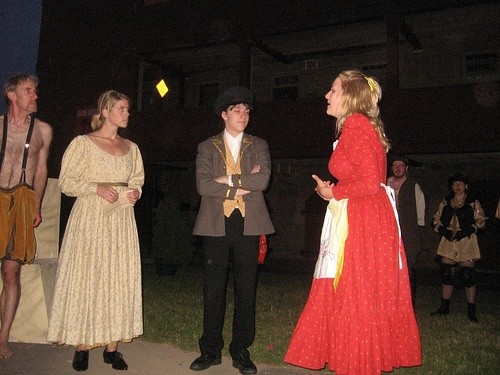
[213,86,254,117]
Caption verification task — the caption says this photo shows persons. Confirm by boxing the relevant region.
[46,89,145,370]
[0,70,52,360]
[282,69,422,375]
[189,85,271,375]
[431,172,486,324]
[386,156,425,310]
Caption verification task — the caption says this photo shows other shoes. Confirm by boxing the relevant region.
[189,350,222,370]
[73,350,90,371]
[103,348,128,370]
[233,349,257,375]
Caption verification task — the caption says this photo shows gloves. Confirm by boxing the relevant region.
[452,224,475,241]
[438,225,453,241]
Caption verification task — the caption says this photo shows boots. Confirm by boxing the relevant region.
[430,298,450,315]
[467,301,478,323]
